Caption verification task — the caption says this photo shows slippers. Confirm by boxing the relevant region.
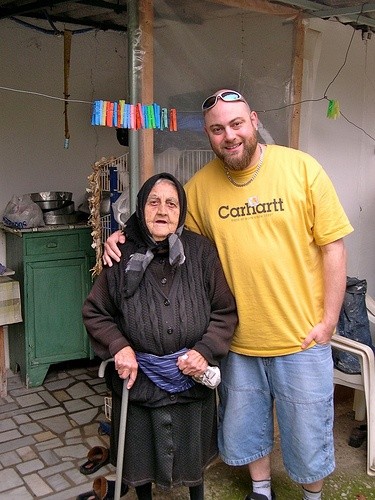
[80,446,111,474]
[77,476,129,500]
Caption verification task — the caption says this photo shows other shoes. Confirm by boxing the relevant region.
[244,488,276,500]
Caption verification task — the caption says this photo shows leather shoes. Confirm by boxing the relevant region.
[348,424,367,448]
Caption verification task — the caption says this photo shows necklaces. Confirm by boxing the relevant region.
[224,142,263,187]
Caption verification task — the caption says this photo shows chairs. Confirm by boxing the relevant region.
[330,294,375,476]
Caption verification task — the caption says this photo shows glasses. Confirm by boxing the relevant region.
[201,91,251,125]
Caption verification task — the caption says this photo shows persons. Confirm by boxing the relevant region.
[82,172,238,500]
[103,89,355,500]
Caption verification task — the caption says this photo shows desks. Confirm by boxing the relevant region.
[0,277,23,398]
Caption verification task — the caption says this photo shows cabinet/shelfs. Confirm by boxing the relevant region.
[0,222,96,389]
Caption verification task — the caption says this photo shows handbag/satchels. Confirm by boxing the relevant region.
[2,194,46,227]
[135,347,199,393]
